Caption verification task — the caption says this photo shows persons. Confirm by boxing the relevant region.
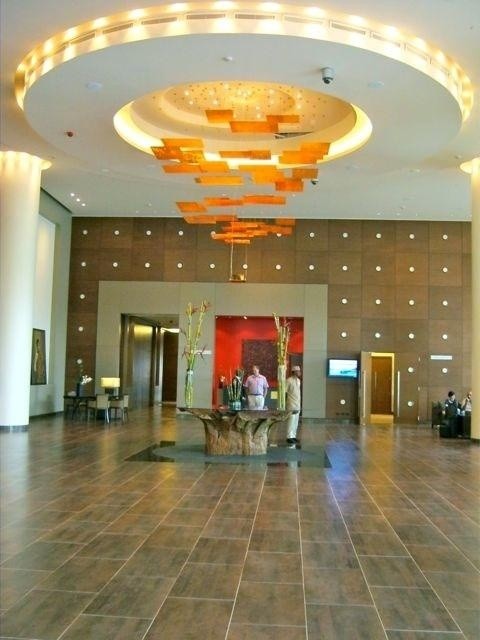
[285,365,302,442]
[444,391,463,437]
[461,391,472,412]
[245,365,269,408]
[218,369,247,410]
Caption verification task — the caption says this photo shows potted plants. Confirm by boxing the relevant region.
[179,297,211,408]
[273,311,290,411]
[222,370,245,410]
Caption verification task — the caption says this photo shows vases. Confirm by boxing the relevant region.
[76,382,82,396]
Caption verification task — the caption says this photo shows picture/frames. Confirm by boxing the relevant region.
[31,328,47,385]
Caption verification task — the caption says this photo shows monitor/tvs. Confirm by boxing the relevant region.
[327,358,359,379]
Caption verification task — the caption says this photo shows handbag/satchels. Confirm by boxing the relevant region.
[440,425,450,437]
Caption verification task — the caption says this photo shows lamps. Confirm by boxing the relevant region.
[151,108,331,245]
[101,377,120,400]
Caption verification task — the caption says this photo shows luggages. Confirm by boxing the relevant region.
[449,414,464,438]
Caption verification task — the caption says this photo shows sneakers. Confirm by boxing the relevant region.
[287,438,300,443]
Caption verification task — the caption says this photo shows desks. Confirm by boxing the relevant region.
[63,394,128,420]
[178,406,299,456]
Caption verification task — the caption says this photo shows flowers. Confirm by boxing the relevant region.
[77,374,92,385]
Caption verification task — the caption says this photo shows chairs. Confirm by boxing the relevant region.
[109,395,130,421]
[87,395,108,423]
[431,400,471,438]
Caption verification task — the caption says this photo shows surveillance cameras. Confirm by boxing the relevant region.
[322,68,334,84]
[311,178,319,185]
[66,130,74,137]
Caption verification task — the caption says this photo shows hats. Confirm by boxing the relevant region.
[291,366,300,372]
